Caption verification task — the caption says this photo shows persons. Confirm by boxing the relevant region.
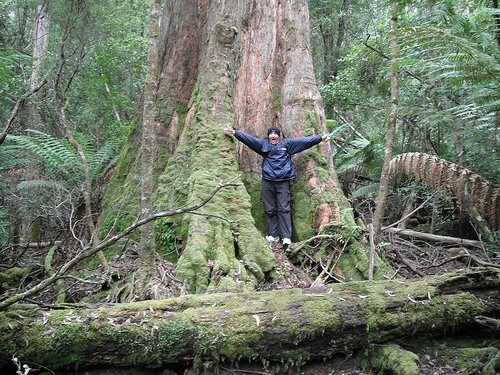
[224,127,330,246]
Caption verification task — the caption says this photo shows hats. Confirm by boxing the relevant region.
[267,126,280,137]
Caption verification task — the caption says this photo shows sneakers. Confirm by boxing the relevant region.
[282,238,291,245]
[266,235,279,243]
